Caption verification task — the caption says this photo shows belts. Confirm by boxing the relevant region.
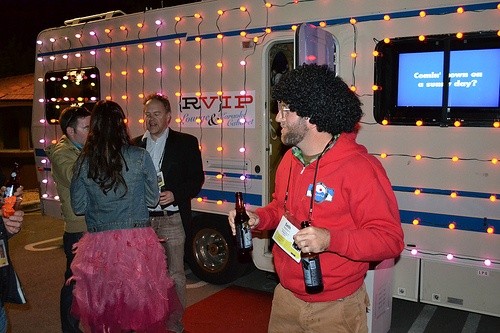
[149,209,178,217]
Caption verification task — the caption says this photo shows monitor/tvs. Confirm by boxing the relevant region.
[388,43,500,119]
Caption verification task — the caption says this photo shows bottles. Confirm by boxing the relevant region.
[4,162,20,199]
[234,192,253,253]
[301,220,324,294]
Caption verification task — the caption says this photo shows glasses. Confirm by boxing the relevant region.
[277,100,292,119]
[65,106,89,129]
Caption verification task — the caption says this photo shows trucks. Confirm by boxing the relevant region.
[30,0,500,319]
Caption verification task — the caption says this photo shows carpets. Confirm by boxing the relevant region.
[182,284,275,333]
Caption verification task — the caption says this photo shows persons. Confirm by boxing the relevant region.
[227,62,405,333]
[44,105,92,333]
[129,93,204,333]
[0,184,24,333]
[69,101,186,333]
